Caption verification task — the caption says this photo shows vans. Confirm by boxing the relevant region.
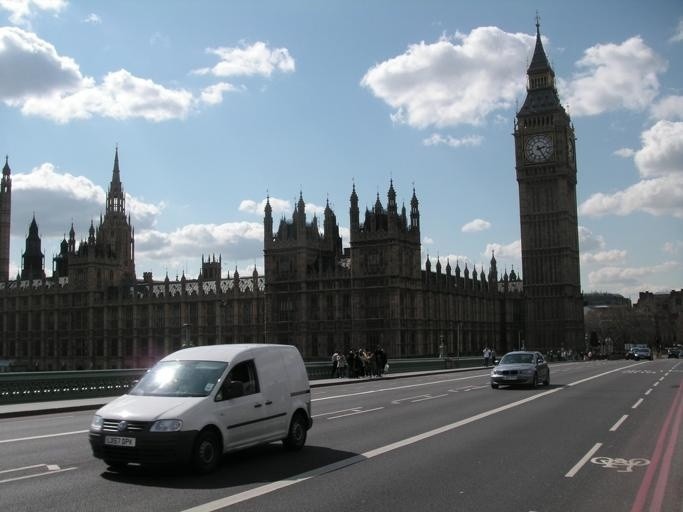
[88,343,313,477]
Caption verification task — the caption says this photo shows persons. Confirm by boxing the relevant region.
[330,344,387,379]
[482,346,491,367]
[222,371,245,400]
[547,346,592,361]
[490,348,496,364]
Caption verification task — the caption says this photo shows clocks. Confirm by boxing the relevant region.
[568,138,576,164]
[524,133,555,163]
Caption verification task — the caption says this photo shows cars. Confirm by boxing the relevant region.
[668,348,680,359]
[624,347,653,360]
[490,351,549,388]
